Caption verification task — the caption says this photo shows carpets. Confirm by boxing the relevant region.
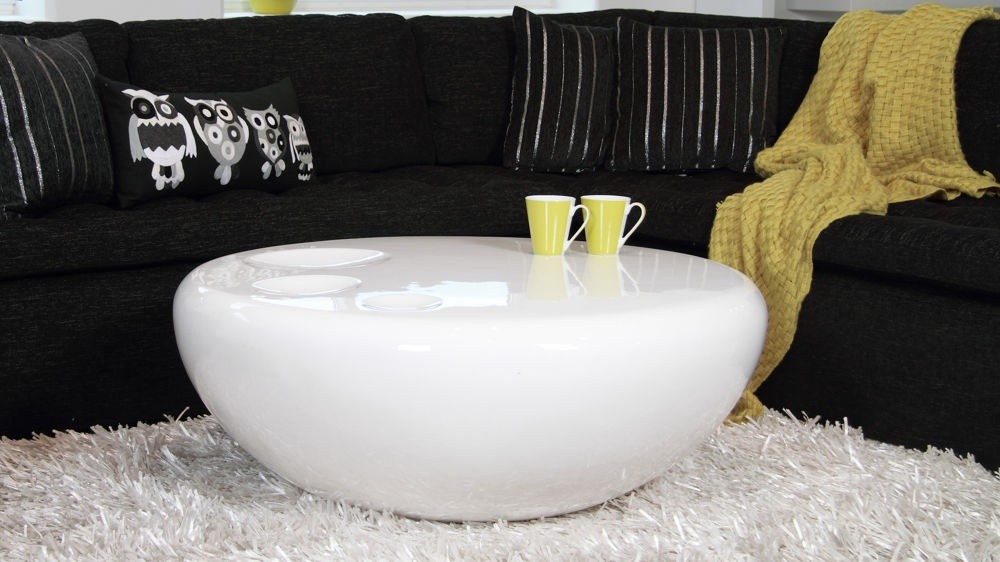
[0,409,1000,561]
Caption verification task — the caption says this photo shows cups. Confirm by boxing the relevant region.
[524,195,589,256]
[581,195,647,254]
[251,0,298,14]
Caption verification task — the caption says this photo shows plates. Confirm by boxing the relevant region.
[359,293,442,309]
[243,248,384,268]
[252,274,362,296]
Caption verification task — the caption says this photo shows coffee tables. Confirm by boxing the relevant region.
[172,236,766,519]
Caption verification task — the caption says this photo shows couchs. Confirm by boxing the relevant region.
[0,8,1000,471]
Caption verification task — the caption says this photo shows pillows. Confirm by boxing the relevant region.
[505,4,616,172]
[607,13,791,175]
[0,31,114,219]
[90,73,315,206]
